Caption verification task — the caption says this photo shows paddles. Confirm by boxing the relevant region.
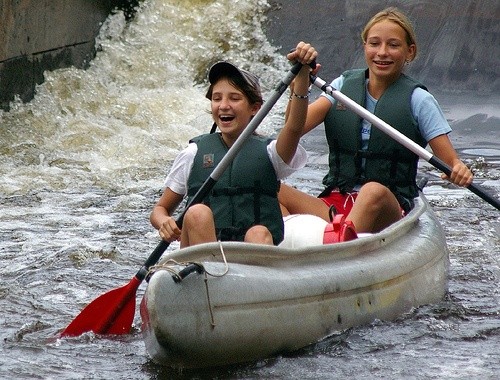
[308,73,500,209]
[60,48,315,338]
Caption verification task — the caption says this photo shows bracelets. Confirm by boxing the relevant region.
[290,96,293,101]
[293,90,311,99]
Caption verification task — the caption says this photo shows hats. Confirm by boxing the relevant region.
[208,60,263,101]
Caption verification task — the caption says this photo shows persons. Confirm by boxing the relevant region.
[277,6,475,234]
[149,40,318,249]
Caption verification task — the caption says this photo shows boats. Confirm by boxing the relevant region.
[139,175,451,362]
